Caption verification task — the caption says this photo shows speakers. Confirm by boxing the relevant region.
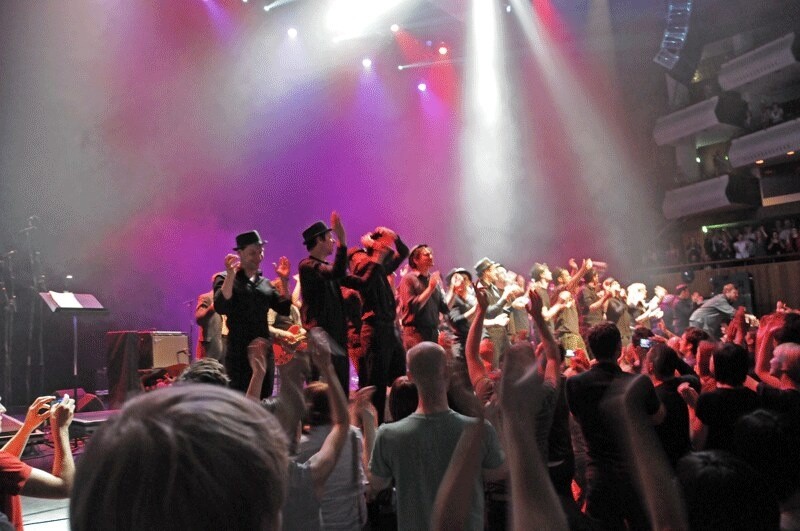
[106,332,191,400]
[53,389,106,413]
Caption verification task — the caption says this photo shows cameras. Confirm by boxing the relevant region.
[639,337,656,348]
[50,399,63,409]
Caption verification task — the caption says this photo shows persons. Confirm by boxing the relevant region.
[69,382,292,531]
[0,211,800,531]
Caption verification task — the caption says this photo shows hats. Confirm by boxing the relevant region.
[302,221,332,245]
[233,230,266,251]
[446,267,472,284]
[409,244,428,269]
[475,257,494,276]
[529,262,547,282]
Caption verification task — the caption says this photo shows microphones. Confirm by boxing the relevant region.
[177,349,188,355]
